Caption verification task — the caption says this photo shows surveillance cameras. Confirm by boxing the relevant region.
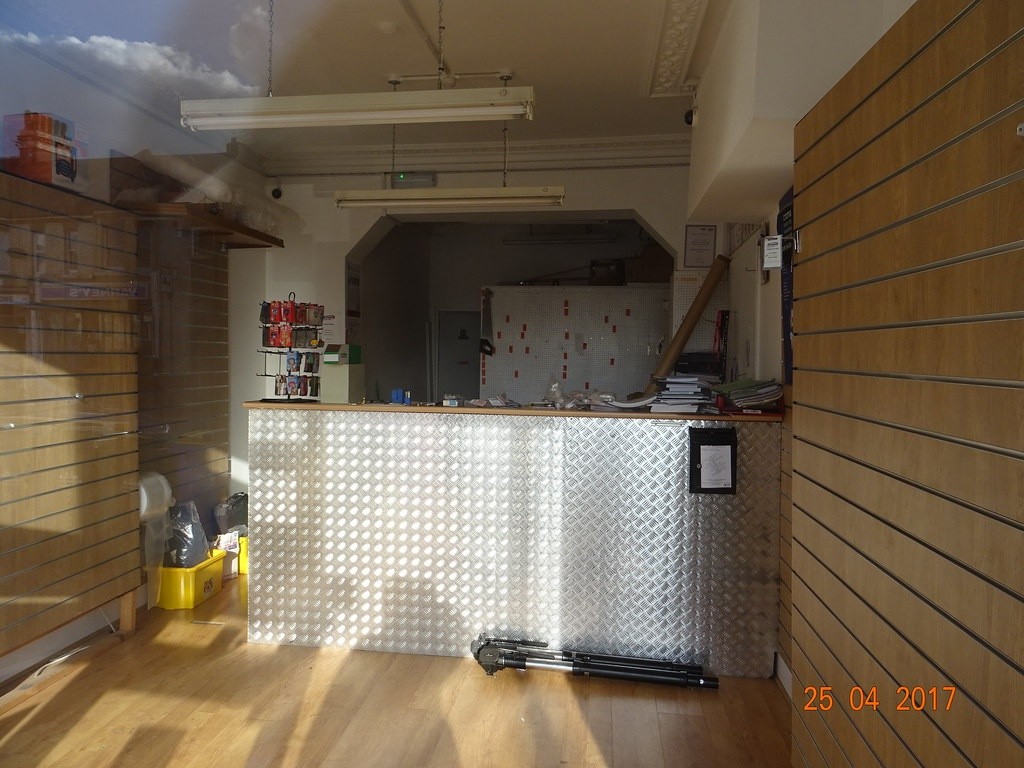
[684,109,694,125]
[272,189,282,199]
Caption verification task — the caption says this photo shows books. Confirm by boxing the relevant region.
[589,375,782,415]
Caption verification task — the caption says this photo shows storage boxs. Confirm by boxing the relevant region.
[0,111,91,195]
[140,547,227,609]
[239,536,249,575]
[323,343,363,365]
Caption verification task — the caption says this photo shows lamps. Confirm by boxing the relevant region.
[334,69,566,210]
[180,0,535,133]
[503,223,617,245]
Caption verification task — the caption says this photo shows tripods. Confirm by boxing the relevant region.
[470,633,721,690]
